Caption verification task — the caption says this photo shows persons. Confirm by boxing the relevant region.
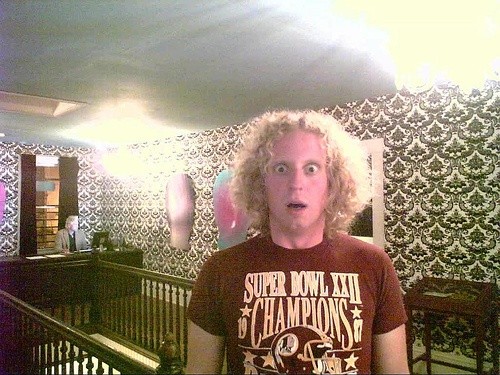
[182,108,413,375]
[55,215,88,254]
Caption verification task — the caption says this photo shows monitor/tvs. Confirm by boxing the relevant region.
[91,232,109,248]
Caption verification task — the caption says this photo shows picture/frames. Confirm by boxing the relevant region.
[342,136,387,252]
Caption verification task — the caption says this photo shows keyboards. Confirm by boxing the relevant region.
[74,249,93,253]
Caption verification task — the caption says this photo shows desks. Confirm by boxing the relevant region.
[400,275,500,375]
[0,242,144,305]
